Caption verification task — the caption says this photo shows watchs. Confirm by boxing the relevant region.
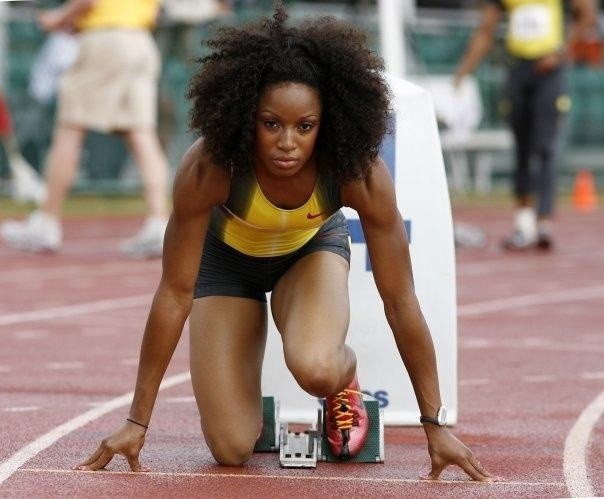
[418,404,446,426]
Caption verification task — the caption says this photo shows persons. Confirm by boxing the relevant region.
[455,0,604,249]
[69,0,497,482]
[0,1,234,254]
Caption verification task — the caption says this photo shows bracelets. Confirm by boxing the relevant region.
[126,416,150,429]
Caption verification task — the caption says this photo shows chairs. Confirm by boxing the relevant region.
[426,71,519,199]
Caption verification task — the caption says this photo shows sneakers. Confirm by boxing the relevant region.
[455,205,554,255]
[4,217,171,255]
[320,374,368,460]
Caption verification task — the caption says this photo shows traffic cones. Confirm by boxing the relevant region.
[574,170,597,211]
